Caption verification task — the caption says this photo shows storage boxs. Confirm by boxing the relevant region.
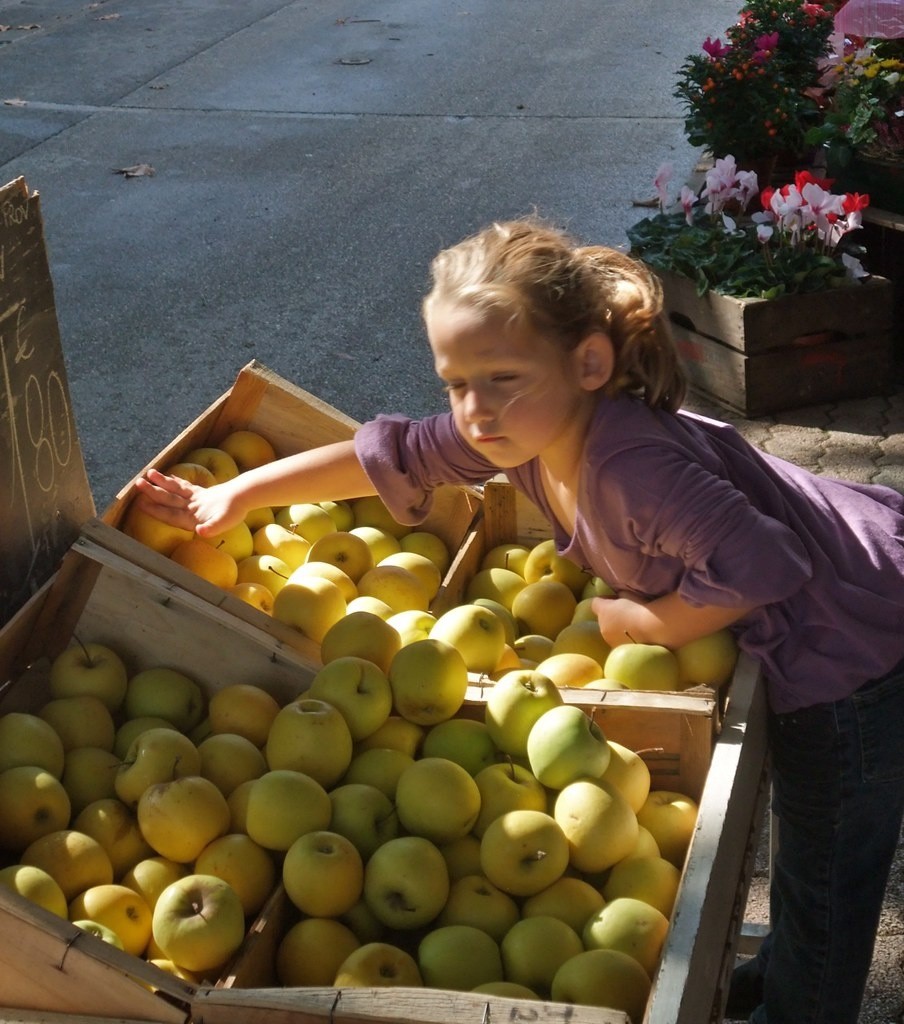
[0,538,324,1023]
[82,355,485,665]
[427,471,772,880]
[187,705,739,1024]
[627,252,904,419]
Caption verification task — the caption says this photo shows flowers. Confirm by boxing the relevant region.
[627,1,904,296]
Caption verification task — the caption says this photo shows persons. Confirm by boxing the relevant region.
[137,221,904,1023]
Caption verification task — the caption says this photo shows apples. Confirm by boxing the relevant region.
[1,431,740,1019]
